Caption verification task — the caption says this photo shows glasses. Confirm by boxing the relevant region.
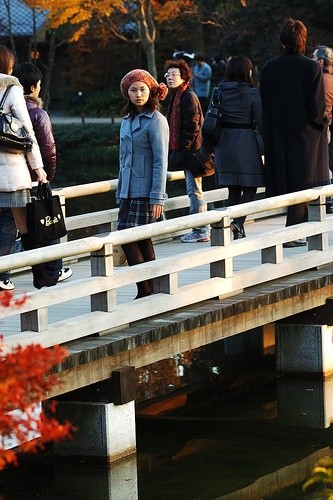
[164,73,180,78]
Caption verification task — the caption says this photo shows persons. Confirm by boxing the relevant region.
[164,19,333,248]
[116,69,170,300]
[0,45,72,290]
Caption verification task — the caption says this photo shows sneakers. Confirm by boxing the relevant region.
[180,231,209,243]
[0,279,14,289]
[58,267,73,282]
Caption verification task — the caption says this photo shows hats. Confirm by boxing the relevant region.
[120,69,168,101]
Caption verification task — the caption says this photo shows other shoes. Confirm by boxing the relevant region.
[230,221,246,240]
[282,241,307,248]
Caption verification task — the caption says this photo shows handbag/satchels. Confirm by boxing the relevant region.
[26,180,68,244]
[202,86,222,143]
[187,147,216,177]
[0,85,33,153]
